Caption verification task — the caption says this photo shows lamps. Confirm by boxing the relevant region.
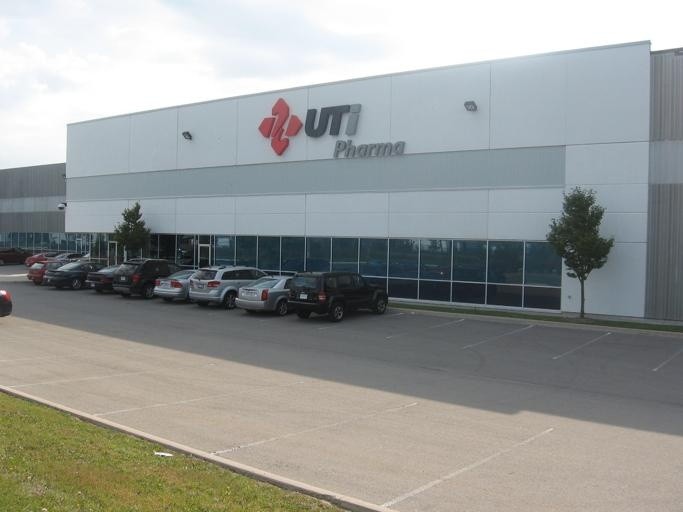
[464,101,477,111]
[182,131,191,140]
[58,202,67,210]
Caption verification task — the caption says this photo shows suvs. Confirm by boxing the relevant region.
[188,265,269,310]
[0,247,33,265]
[287,270,388,323]
[112,257,183,301]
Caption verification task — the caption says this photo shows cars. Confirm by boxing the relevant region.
[153,269,200,303]
[85,265,120,294]
[234,275,296,316]
[0,289,13,318]
[25,252,108,292]
